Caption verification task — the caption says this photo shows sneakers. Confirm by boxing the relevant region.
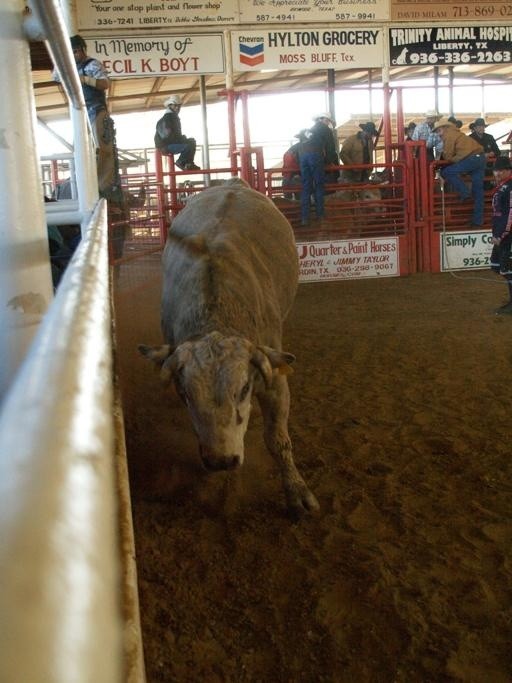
[301,215,324,225]
[175,158,201,170]
[496,301,512,314]
[460,195,482,227]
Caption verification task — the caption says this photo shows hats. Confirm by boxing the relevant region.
[70,35,87,50]
[404,122,416,135]
[431,114,462,132]
[488,156,511,169]
[294,128,311,138]
[469,118,489,130]
[164,94,184,106]
[359,122,379,136]
[312,111,335,124]
[422,108,443,122]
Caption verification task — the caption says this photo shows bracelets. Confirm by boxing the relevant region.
[85,76,96,88]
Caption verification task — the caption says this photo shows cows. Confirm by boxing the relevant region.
[271,184,388,241]
[136,177,320,514]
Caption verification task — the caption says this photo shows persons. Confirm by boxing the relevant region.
[42,175,82,290]
[404,109,501,231]
[108,174,147,276]
[176,180,195,209]
[52,35,117,202]
[154,96,202,170]
[281,113,379,227]
[489,157,512,315]
